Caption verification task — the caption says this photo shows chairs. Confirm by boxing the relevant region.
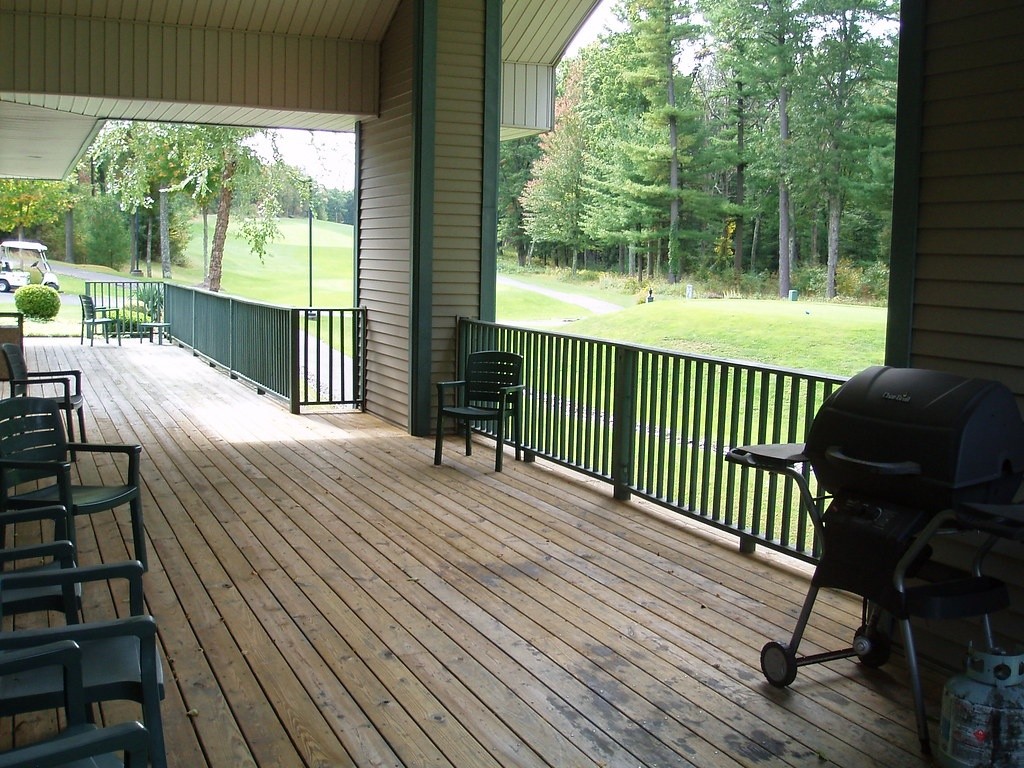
[1,560,168,768]
[3,342,88,462]
[0,397,149,573]
[434,350,525,472]
[79,295,121,346]
[0,505,82,627]
[1,640,150,768]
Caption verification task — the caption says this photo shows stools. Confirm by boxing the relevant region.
[141,322,172,345]
